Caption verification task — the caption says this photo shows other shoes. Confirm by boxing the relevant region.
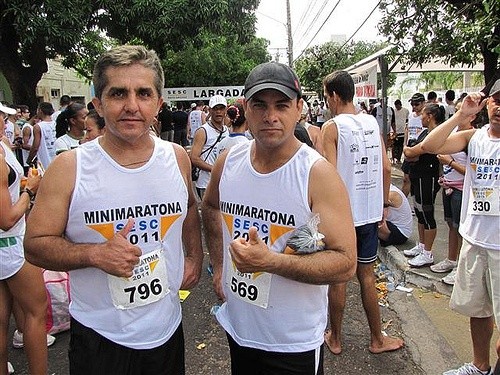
[411,209,415,216]
[207,263,213,277]
[397,162,401,164]
[393,160,396,165]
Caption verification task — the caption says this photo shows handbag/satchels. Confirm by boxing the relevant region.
[42,269,71,335]
[192,166,197,181]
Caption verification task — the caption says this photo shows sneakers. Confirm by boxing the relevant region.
[13,330,56,348]
[408,253,434,267]
[404,245,425,257]
[442,361,493,375]
[430,259,458,273]
[442,268,458,285]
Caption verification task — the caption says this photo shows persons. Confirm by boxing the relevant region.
[23,45,203,375]
[201,61,357,375]
[358,90,487,286]
[0,93,107,375]
[158,93,329,200]
[322,70,403,353]
[421,79,500,375]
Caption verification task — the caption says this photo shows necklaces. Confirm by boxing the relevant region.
[211,121,227,133]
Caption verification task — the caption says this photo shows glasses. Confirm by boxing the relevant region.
[411,102,422,106]
[25,112,30,113]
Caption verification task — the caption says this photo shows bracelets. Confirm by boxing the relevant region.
[20,188,34,201]
[448,159,455,165]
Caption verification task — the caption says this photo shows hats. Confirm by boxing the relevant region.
[489,79,500,96]
[209,95,227,108]
[0,102,16,115]
[243,61,302,103]
[408,93,425,102]
[191,103,196,108]
